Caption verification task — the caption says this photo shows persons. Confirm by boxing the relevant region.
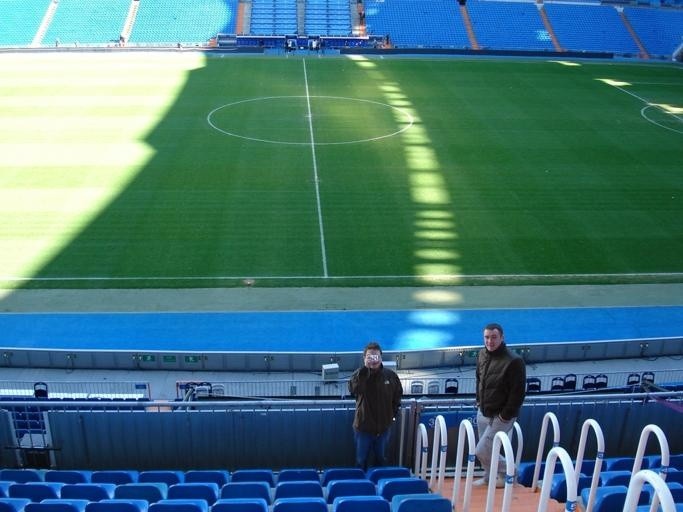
[120,35,124,47]
[284,39,292,53]
[472,324,527,488]
[373,38,377,48]
[348,342,402,471]
[359,12,365,24]
[312,38,325,57]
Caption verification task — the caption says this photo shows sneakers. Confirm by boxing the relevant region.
[474,473,506,487]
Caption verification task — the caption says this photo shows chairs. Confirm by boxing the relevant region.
[517,455,683,511]
[3,399,148,437]
[0,466,450,512]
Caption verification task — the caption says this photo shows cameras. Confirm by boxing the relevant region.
[368,355,379,363]
